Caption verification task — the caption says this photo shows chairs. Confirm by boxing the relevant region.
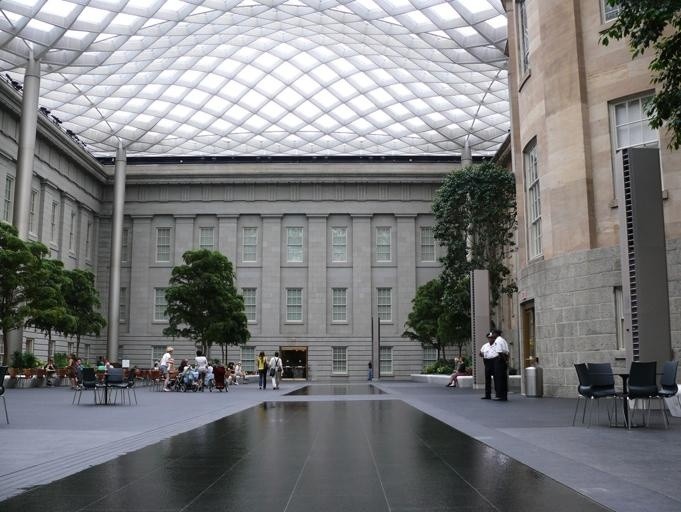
[572,360,679,429]
[152,368,177,391]
[72,368,138,405]
[0,366,9,424]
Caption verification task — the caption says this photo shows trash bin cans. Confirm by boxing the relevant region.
[525,366,543,398]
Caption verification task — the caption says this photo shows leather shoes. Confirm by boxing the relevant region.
[449,385,455,387]
[493,396,508,401]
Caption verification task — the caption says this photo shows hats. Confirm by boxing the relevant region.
[486,332,494,337]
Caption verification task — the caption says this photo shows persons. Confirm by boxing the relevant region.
[368,360,373,381]
[154,345,251,392]
[445,357,466,387]
[42,345,138,390]
[453,357,461,374]
[491,329,511,402]
[256,351,270,389]
[268,351,284,390]
[480,332,501,399]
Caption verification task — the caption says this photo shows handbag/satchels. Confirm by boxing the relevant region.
[270,368,276,376]
[264,358,268,369]
